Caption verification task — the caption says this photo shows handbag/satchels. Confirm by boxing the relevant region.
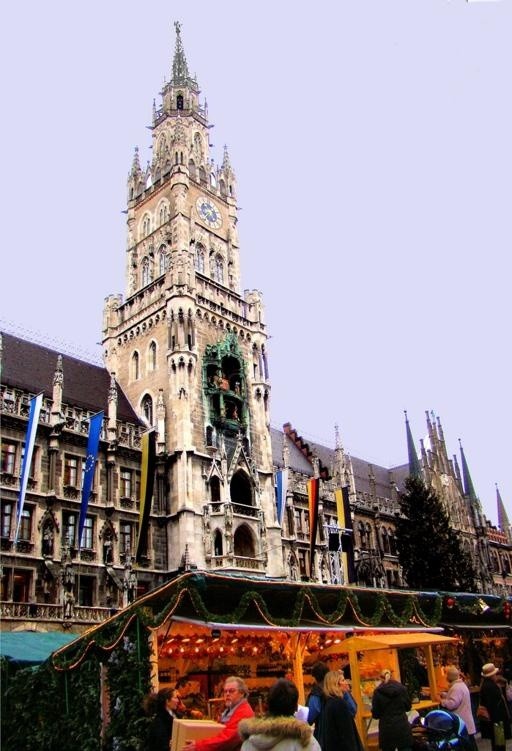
[476,705,490,721]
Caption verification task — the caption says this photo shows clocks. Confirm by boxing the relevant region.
[195,196,223,230]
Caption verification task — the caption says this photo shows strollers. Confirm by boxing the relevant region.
[412,709,471,751]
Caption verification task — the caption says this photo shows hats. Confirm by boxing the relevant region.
[481,663,500,679]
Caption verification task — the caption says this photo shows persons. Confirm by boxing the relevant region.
[371,664,509,750]
[148,664,365,750]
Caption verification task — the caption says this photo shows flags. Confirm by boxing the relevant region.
[275,470,286,524]
[76,412,106,547]
[12,394,41,521]
[305,478,319,577]
[334,486,355,583]
[133,431,155,565]
